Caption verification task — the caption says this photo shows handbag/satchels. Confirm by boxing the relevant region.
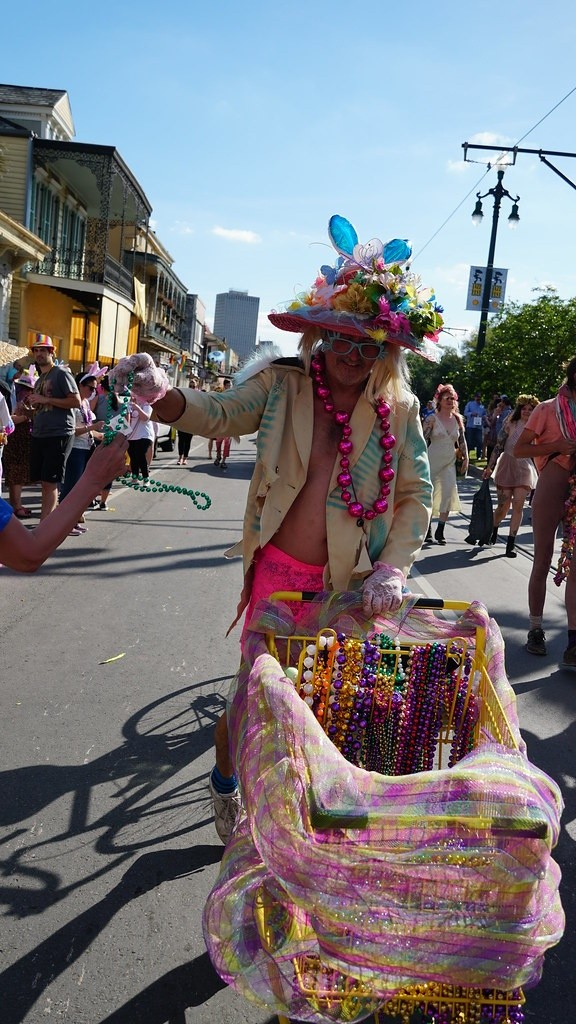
[469,475,494,541]
[455,446,468,480]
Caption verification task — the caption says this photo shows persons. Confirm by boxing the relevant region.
[464,392,513,470]
[422,400,436,418]
[0,432,129,572]
[514,351,576,666]
[108,328,435,846]
[422,384,469,544]
[177,381,196,465]
[482,394,542,559]
[208,379,232,468]
[0,333,154,536]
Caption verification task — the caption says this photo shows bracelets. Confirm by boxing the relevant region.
[85,426,88,431]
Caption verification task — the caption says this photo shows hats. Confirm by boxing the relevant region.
[30,334,54,350]
[267,214,446,363]
[475,392,482,397]
[0,340,29,367]
[14,364,38,388]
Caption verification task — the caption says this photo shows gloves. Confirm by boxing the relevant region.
[109,351,172,406]
[359,560,405,618]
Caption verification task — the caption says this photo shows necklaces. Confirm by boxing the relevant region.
[80,399,91,425]
[311,348,396,522]
[103,370,212,511]
[303,633,483,776]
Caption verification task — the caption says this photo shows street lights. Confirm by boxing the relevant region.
[469,148,521,366]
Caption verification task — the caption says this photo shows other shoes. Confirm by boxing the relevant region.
[214,457,221,466]
[221,462,228,469]
[86,500,100,509]
[130,478,139,485]
[68,528,82,535]
[183,457,188,465]
[74,523,88,531]
[177,457,183,465]
[143,477,150,487]
[100,502,108,510]
[13,506,31,516]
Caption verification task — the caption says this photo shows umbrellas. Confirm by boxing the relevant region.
[209,351,224,361]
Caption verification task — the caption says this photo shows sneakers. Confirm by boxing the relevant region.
[209,769,242,845]
[561,646,576,666]
[527,628,546,655]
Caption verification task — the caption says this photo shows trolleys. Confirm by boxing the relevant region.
[248,589,551,1024]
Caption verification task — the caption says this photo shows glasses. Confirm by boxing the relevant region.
[320,330,389,360]
[441,395,455,400]
[81,383,97,392]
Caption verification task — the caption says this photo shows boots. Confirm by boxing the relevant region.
[434,524,446,544]
[489,526,498,544]
[424,522,434,544]
[506,536,517,558]
